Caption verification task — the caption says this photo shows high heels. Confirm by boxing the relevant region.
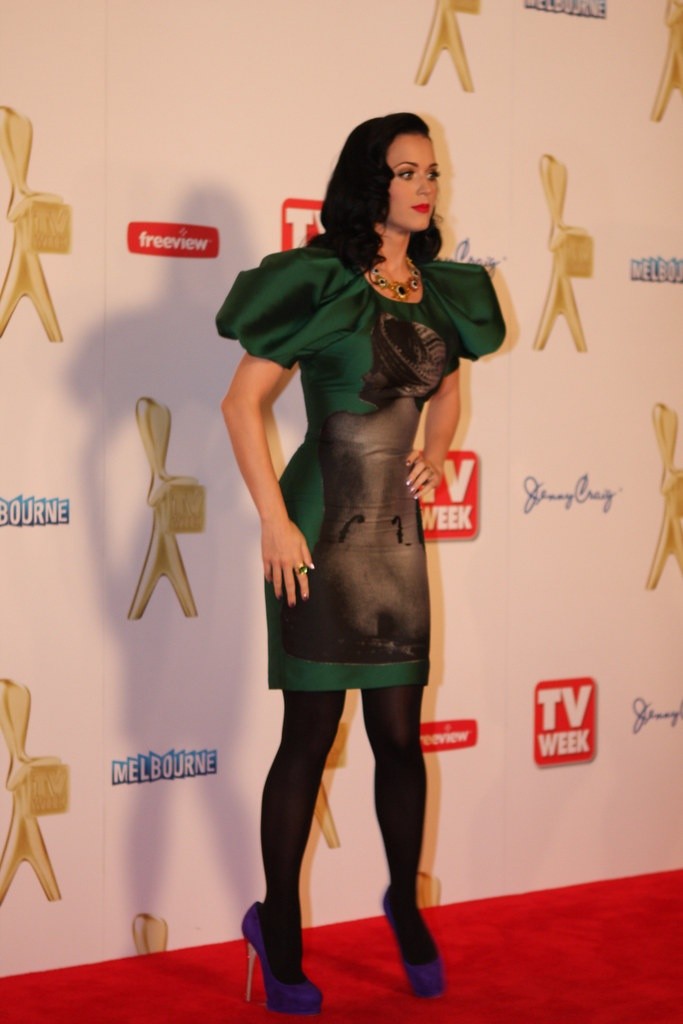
[382,883,445,997]
[241,901,323,1014]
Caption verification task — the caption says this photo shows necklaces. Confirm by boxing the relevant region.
[367,252,420,299]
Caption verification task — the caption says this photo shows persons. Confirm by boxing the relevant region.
[216,111,508,1015]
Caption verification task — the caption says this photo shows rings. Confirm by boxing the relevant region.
[294,565,307,576]
